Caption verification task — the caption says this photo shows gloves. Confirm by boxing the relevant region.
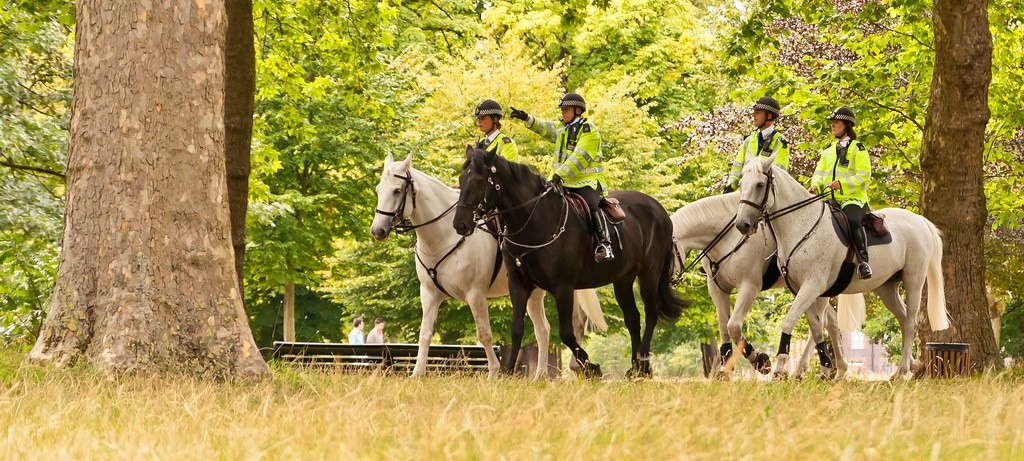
[723,185,734,194]
[551,174,561,186]
[510,106,528,120]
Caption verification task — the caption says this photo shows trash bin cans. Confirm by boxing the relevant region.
[924,341,971,379]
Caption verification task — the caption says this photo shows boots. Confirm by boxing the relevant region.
[593,207,613,258]
[855,226,872,279]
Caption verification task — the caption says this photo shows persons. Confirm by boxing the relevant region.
[699,94,790,272]
[366,316,385,344]
[349,317,365,345]
[509,92,617,261]
[448,98,519,188]
[807,105,872,278]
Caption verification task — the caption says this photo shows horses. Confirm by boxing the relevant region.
[369,148,610,378]
[736,141,950,383]
[452,141,696,384]
[670,185,848,382]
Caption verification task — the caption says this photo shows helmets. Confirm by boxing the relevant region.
[475,99,502,115]
[826,107,856,124]
[558,93,585,108]
[752,97,781,115]
